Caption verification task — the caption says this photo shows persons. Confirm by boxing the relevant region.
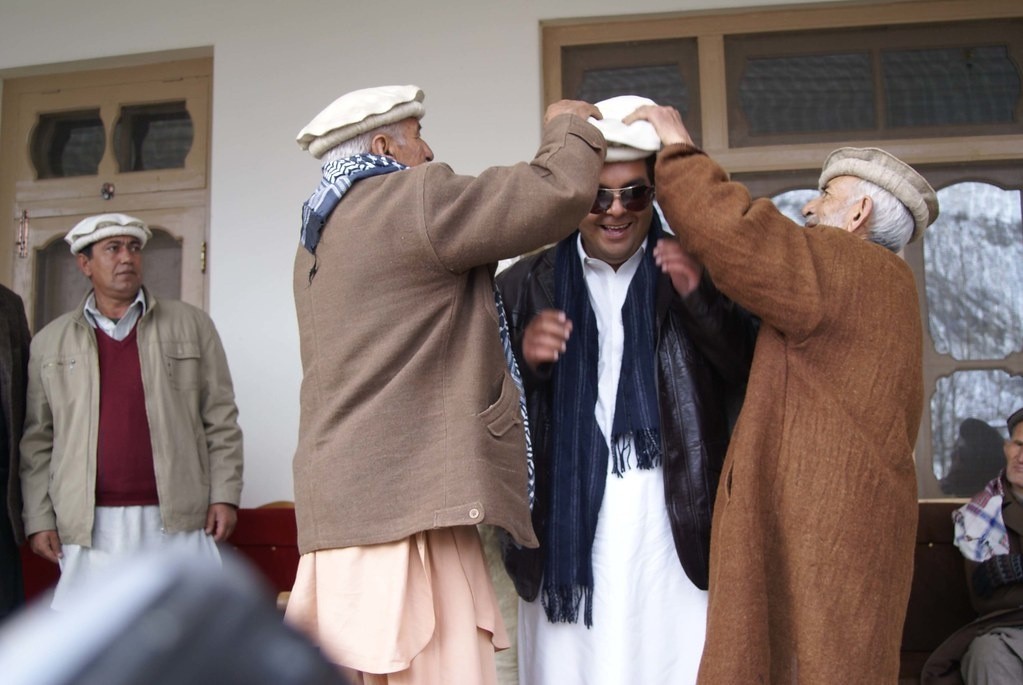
[24,212,245,605]
[952,407,1023,685]
[286,84,609,685]
[495,95,760,685]
[621,103,940,685]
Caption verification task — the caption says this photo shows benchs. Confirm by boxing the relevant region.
[221,500,302,625]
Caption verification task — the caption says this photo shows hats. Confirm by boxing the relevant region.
[65,213,152,256]
[960,418,1004,458]
[581,95,663,164]
[296,85,425,159]
[819,145,940,244]
[1007,406,1023,435]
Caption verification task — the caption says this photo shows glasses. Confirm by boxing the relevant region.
[585,185,656,214]
